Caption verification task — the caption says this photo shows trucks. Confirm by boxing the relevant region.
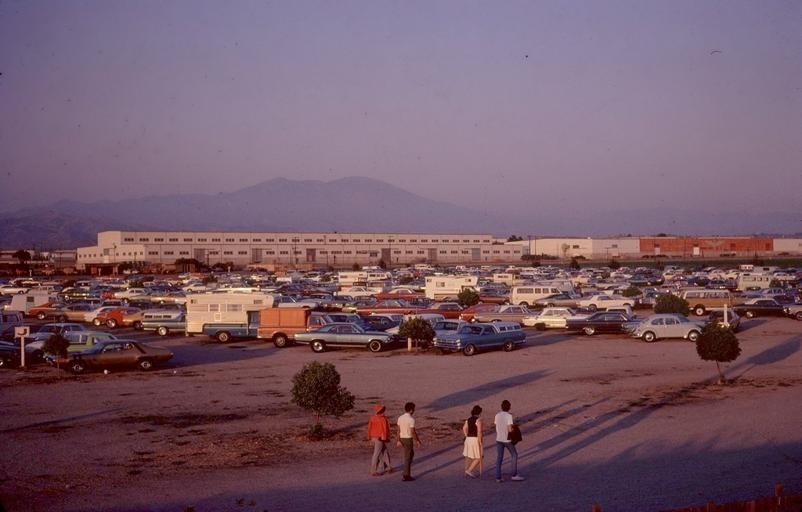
[255,306,330,348]
[184,293,283,336]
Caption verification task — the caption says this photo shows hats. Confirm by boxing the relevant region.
[374,405,384,413]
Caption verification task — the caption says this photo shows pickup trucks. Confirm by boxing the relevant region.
[202,310,260,344]
[139,314,186,337]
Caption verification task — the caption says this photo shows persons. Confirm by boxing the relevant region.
[395,402,422,482]
[460,404,486,478]
[367,402,394,477]
[494,400,526,484]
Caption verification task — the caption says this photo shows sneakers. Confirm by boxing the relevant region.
[465,469,476,479]
[511,474,525,481]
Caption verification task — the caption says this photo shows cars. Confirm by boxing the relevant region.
[563,310,641,337]
[626,313,707,341]
[14,322,87,346]
[26,339,67,370]
[2,264,802,328]
[431,323,527,352]
[2,341,22,368]
[64,331,119,354]
[69,339,174,369]
[293,323,397,353]
